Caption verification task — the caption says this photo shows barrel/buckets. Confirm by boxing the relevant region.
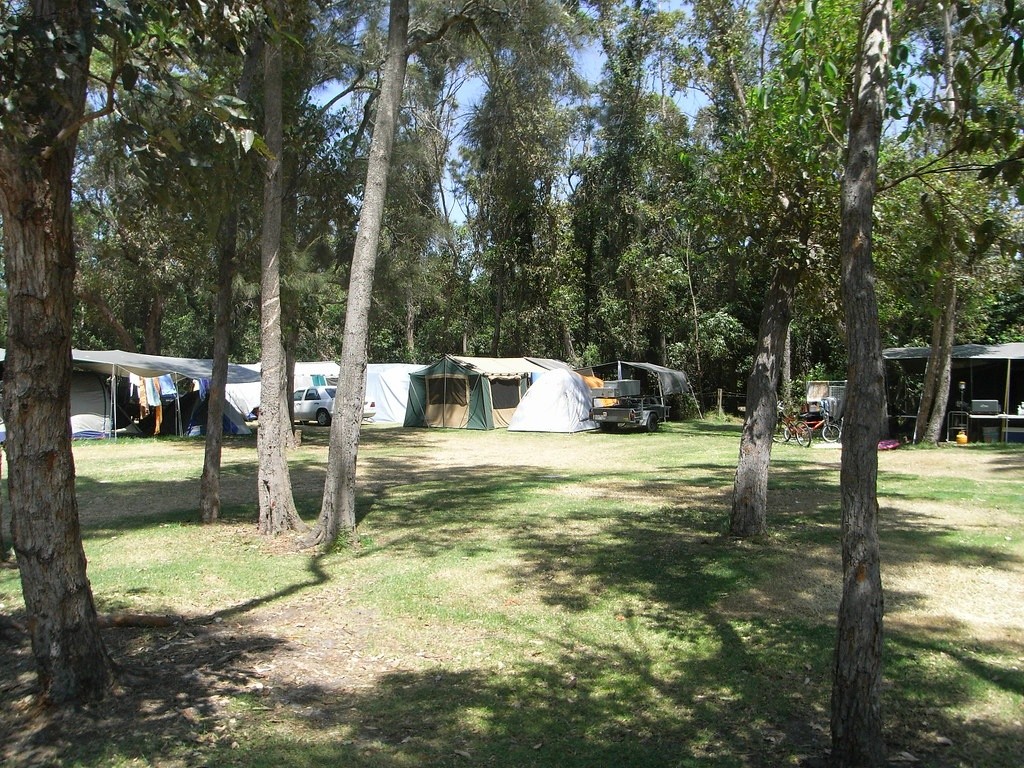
[982,426,1001,444]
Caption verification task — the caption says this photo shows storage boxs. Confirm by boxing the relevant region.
[591,379,640,407]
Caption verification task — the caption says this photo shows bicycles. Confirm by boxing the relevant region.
[784,405,842,444]
[772,399,812,448]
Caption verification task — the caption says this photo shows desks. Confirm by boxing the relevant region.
[968,414,1024,444]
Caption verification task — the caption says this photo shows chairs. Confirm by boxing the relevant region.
[947,411,968,443]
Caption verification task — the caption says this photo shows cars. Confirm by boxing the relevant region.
[292,386,376,427]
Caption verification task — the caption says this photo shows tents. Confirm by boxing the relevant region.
[883,343,1024,443]
[0,348,690,440]
